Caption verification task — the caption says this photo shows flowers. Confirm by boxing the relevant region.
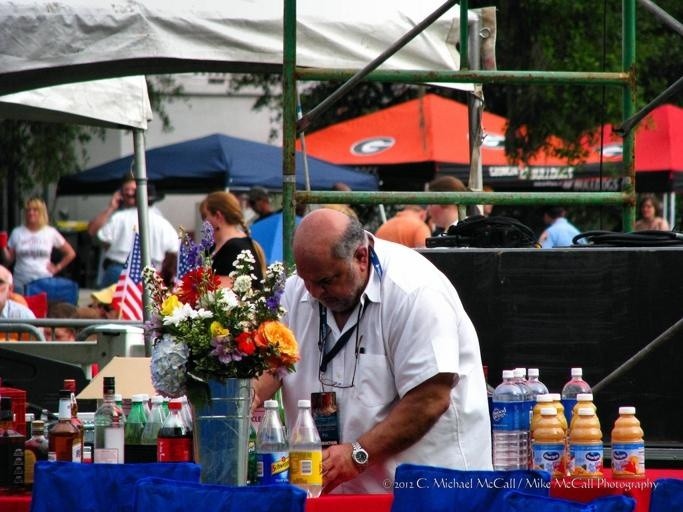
[140,221,299,406]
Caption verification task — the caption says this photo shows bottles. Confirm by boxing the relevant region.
[24,371,195,491]
[482,364,605,479]
[287,400,323,498]
[248,420,256,483]
[254,400,290,483]
[611,405,648,478]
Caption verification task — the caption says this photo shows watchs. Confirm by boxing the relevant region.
[350,441,369,475]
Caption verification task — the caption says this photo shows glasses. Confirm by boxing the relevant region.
[317,329,363,388]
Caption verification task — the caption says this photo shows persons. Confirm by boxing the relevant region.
[247,209,494,496]
[635,195,669,232]
[536,206,590,248]
[0,176,494,342]
